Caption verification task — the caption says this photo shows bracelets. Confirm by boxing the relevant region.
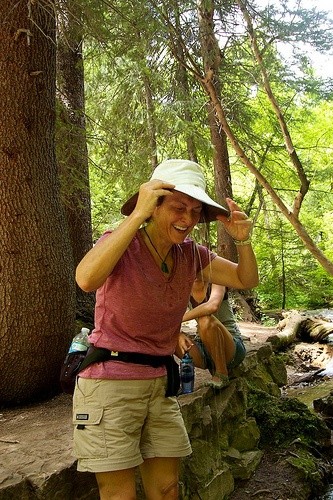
[232,233,252,246]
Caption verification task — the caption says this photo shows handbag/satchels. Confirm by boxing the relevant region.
[61,352,84,393]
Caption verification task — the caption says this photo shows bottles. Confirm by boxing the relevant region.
[68,327,89,352]
[179,352,194,393]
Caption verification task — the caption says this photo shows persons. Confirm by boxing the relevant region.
[174,277,247,386]
[73,160,258,500]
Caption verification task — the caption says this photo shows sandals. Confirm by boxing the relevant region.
[204,374,230,390]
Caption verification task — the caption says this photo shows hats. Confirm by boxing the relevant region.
[121,159,230,224]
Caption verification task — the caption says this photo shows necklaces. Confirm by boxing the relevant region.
[144,226,174,274]
